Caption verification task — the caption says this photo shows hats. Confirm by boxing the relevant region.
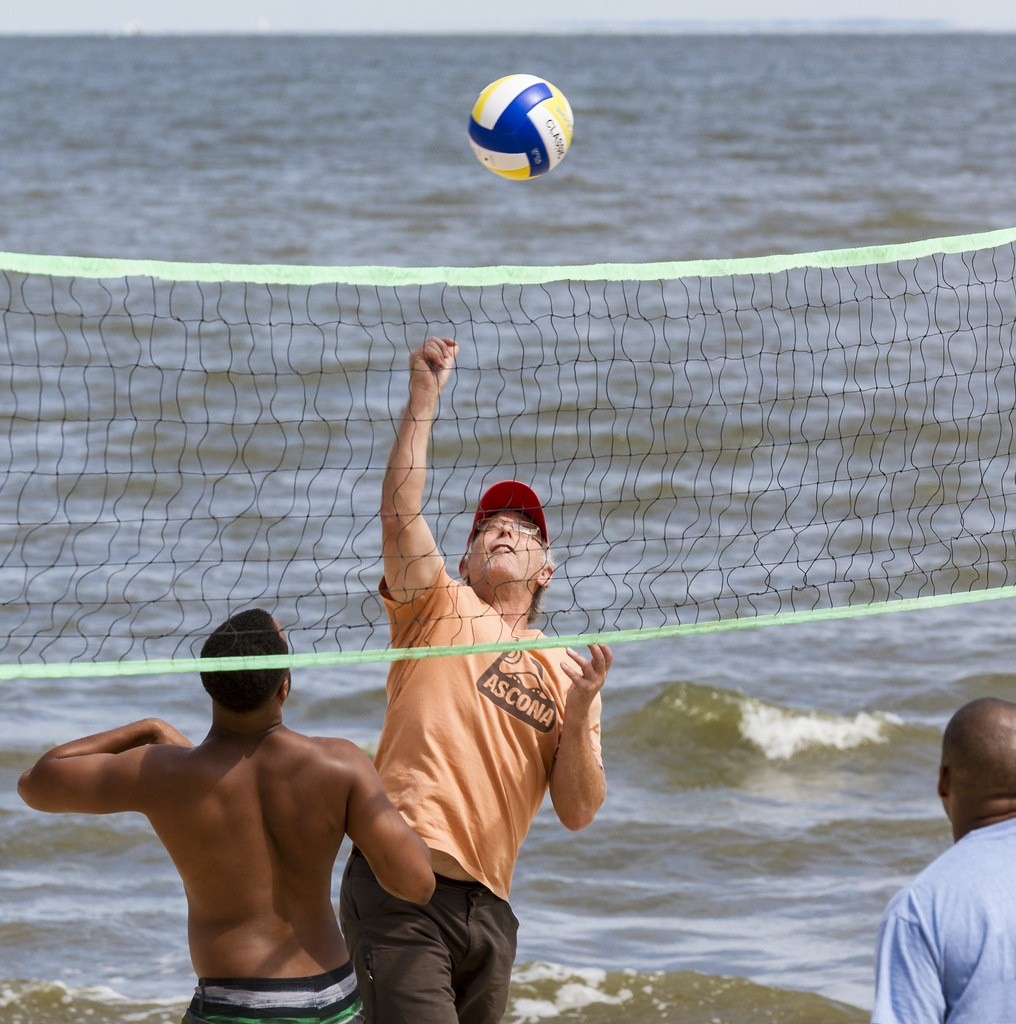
[466,480,548,548]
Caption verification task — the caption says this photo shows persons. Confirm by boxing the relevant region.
[339,338,612,1024]
[17,609,435,1024]
[869,697,1016,1024]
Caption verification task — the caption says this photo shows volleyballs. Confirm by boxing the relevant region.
[465,73,574,182]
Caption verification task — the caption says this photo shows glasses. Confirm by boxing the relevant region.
[475,518,542,544]
